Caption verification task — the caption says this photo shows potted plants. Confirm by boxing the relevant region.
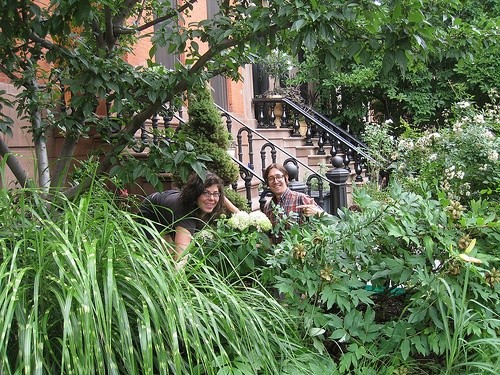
[260,48,290,128]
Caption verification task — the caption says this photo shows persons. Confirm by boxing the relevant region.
[137,170,241,273]
[255,164,334,248]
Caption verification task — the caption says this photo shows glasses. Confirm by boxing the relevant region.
[267,174,286,183]
[201,191,221,197]
